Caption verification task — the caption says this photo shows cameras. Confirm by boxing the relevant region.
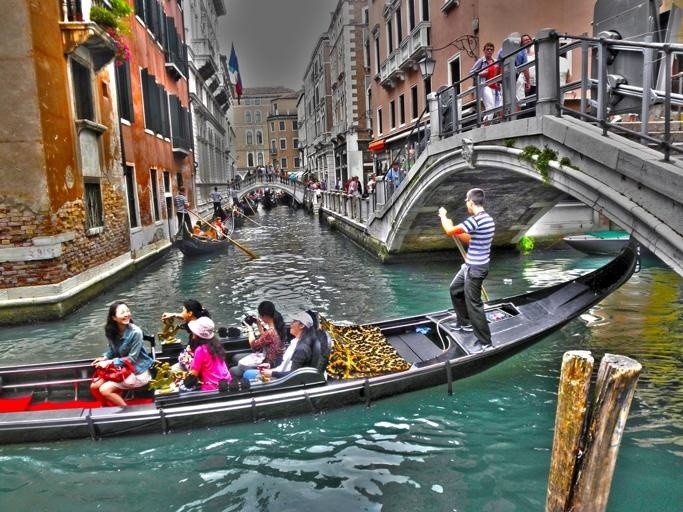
[242,315,256,327]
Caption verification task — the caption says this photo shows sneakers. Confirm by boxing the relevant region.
[468,339,495,353]
[450,322,473,332]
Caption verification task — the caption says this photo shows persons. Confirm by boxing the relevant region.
[468,32,570,121]
[338,150,417,197]
[305,178,326,190]
[162,298,313,394]
[90,302,154,406]
[175,162,295,239]
[439,188,495,354]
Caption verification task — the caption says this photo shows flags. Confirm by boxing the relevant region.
[228,41,243,105]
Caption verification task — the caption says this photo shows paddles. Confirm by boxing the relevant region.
[187,209,258,258]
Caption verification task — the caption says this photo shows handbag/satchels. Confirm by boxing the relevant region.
[92,356,137,383]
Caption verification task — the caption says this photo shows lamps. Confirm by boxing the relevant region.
[417,34,480,81]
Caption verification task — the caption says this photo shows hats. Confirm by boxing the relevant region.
[188,317,215,340]
[288,311,313,329]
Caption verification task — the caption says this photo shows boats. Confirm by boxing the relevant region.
[1,227,643,449]
[560,233,635,257]
[172,187,282,261]
